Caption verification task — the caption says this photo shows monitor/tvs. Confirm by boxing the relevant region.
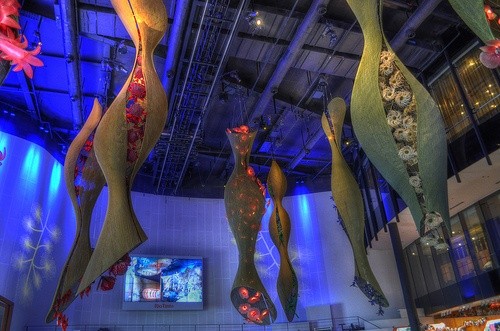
[121,254,204,312]
